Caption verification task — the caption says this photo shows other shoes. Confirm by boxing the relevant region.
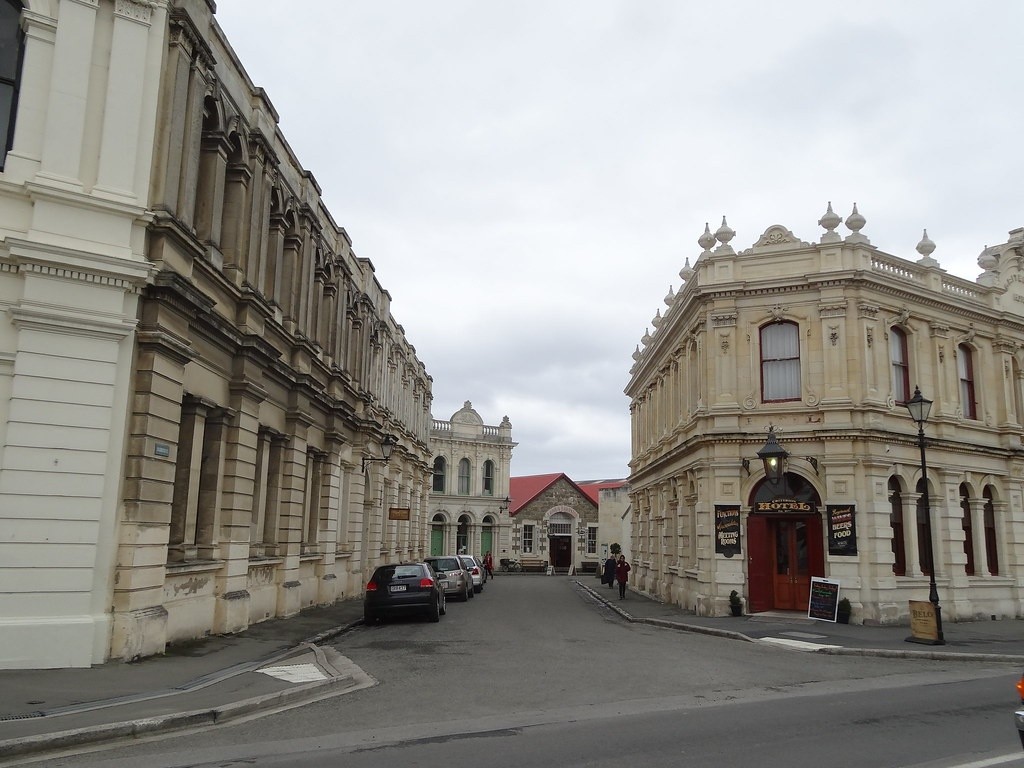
[608,586,613,589]
[491,575,493,579]
[619,595,623,600]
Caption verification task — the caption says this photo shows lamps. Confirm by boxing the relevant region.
[756,425,789,489]
[362,433,395,473]
[499,496,511,514]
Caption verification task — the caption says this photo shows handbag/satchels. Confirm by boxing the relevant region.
[601,574,608,585]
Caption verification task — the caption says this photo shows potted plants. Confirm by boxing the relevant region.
[837,597,852,624]
[728,590,743,617]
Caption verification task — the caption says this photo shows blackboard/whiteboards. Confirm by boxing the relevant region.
[808,576,840,623]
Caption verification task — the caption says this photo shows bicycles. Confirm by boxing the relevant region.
[500,558,524,572]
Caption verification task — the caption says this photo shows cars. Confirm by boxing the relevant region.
[422,555,476,602]
[364,562,448,626]
[455,555,488,594]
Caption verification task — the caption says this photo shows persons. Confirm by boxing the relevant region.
[604,555,617,589]
[615,555,631,599]
[483,551,494,579]
[458,546,464,555]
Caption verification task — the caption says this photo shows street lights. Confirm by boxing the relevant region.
[903,385,949,645]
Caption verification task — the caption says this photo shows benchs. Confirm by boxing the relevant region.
[581,561,599,573]
[520,559,544,572]
